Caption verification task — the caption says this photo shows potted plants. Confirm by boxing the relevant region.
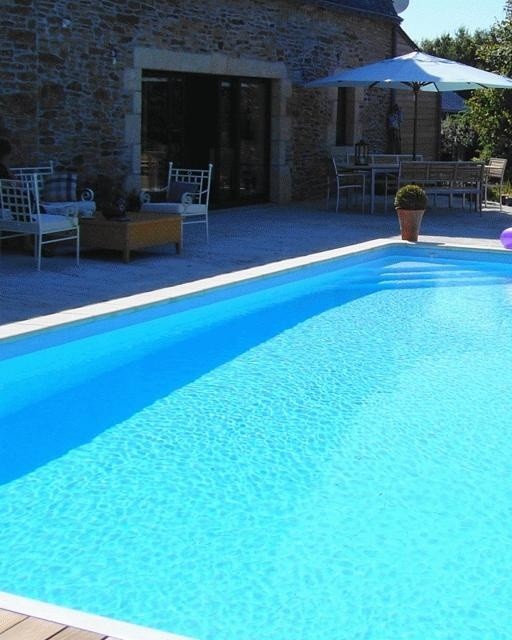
[395,182,425,243]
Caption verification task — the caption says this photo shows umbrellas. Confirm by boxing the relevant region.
[304,48,511,160]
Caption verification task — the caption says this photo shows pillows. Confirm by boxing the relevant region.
[166,182,199,203]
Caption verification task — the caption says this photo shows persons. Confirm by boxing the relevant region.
[0,140,57,258]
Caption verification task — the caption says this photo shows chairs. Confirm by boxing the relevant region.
[3,163,93,272]
[329,152,508,216]
[139,162,214,253]
